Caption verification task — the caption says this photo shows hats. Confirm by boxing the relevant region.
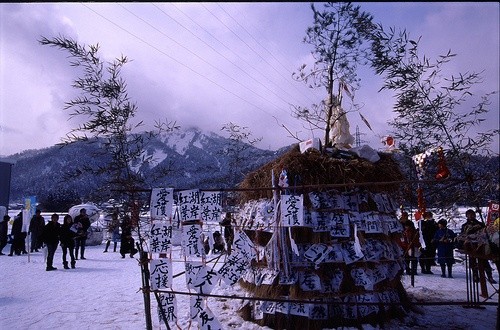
[400,212,409,216]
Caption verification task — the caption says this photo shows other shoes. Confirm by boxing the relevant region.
[474,274,480,282]
[45,266,57,271]
[488,277,497,284]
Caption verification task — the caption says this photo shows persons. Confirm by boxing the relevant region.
[59,214,77,269]
[120,230,138,258]
[8,212,27,256]
[104,212,119,253]
[204,230,226,254]
[397,210,421,275]
[121,215,134,235]
[418,211,439,274]
[434,219,456,278]
[0,215,11,255]
[43,214,61,271]
[219,212,235,256]
[28,208,45,253]
[74,208,90,260]
[461,209,498,284]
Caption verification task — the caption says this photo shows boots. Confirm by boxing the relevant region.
[71,261,77,269]
[62,261,70,269]
